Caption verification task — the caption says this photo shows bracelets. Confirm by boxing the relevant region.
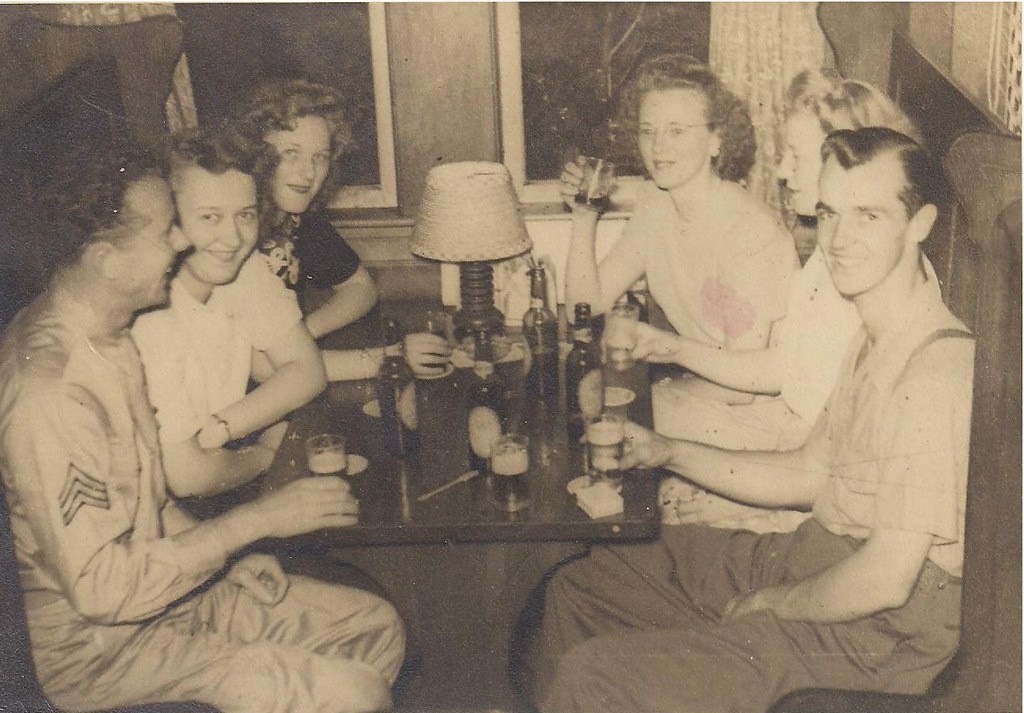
[357,344,392,384]
[211,413,234,441]
[251,437,278,460]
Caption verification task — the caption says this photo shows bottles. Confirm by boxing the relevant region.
[565,303,604,444]
[466,328,508,472]
[521,268,559,395]
[376,317,422,458]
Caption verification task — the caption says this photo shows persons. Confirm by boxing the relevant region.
[599,68,923,532]
[558,54,801,407]
[528,127,977,713]
[0,140,408,712]
[225,75,453,388]
[124,122,329,500]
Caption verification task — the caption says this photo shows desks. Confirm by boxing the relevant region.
[252,256,660,713]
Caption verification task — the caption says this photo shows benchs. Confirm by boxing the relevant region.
[765,435,994,713]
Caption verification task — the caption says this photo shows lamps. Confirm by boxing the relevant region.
[408,160,534,369]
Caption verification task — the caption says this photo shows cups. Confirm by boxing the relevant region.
[304,433,349,481]
[575,158,616,213]
[490,434,529,512]
[585,411,625,494]
[415,307,450,371]
[605,302,638,371]
